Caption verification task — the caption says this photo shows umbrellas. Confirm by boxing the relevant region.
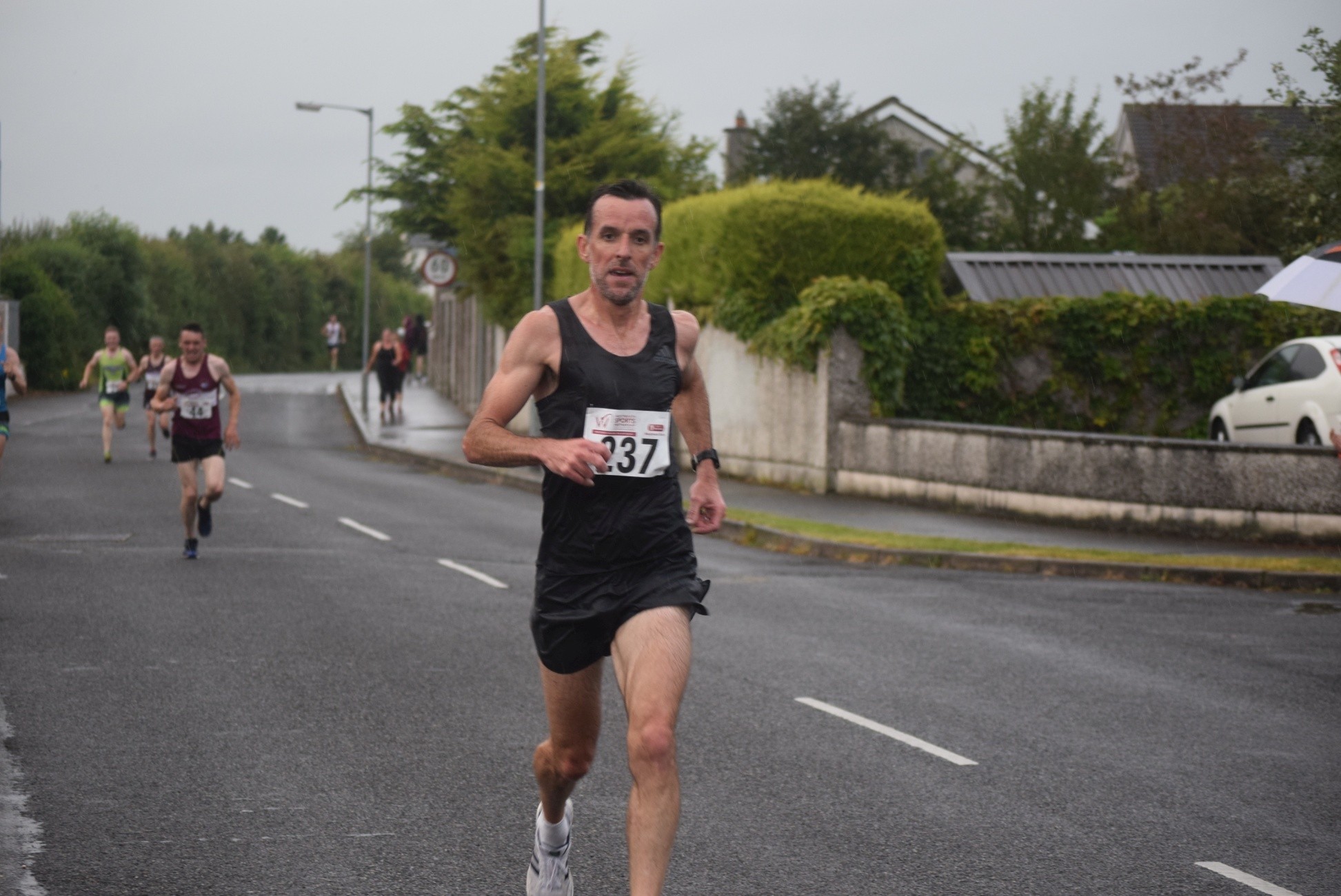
[1255,239,1341,313]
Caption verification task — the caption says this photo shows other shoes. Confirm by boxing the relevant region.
[149,450,157,459]
[196,496,212,537]
[182,537,199,559]
[162,429,170,438]
[104,454,111,463]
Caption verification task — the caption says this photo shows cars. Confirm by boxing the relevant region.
[1207,335,1339,447]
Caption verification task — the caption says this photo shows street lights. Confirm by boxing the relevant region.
[295,100,375,416]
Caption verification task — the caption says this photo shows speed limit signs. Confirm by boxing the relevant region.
[422,250,460,287]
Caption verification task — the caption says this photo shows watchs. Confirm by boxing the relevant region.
[691,448,720,472]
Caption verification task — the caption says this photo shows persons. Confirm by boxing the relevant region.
[0,338,27,458]
[118,335,178,462]
[465,180,724,896]
[365,312,431,422]
[80,327,140,463]
[149,322,240,558]
[322,314,347,373]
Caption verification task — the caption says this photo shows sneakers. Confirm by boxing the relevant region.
[526,797,575,896]
